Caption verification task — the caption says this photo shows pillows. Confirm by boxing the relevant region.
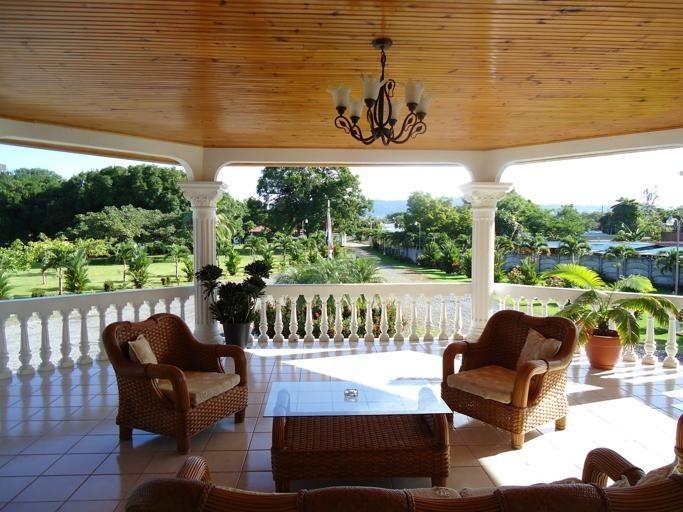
[515,326,563,375]
[125,332,160,384]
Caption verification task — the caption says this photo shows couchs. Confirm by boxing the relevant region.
[124,414,683,511]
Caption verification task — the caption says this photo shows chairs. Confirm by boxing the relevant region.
[102,312,249,453]
[439,311,578,448]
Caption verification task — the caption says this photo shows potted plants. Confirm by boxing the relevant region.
[539,262,682,372]
[193,260,275,351]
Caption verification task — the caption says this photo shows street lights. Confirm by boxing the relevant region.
[415,221,421,266]
[665,215,683,295]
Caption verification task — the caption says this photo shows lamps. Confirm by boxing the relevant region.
[326,40,430,147]
